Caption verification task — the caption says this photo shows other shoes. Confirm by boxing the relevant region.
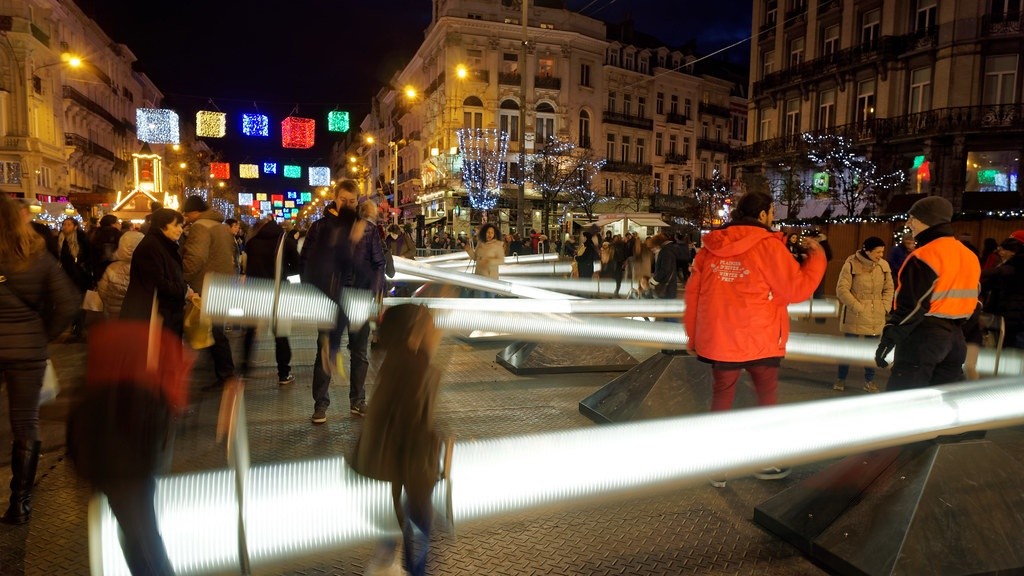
[202,375,237,392]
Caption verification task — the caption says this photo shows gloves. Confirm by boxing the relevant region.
[875,350,888,368]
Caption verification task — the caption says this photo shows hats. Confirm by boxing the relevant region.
[184,195,208,212]
[908,195,953,226]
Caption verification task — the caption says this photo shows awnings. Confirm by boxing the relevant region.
[582,218,620,227]
[630,218,671,227]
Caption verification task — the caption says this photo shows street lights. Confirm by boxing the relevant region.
[367,137,398,225]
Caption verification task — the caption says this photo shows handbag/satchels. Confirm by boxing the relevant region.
[652,284,667,297]
[83,287,103,311]
[183,296,216,350]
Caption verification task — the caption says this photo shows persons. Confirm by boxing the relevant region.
[241,179,419,427]
[785,229,834,325]
[874,195,1024,394]
[1,193,84,526]
[624,228,702,357]
[682,194,827,488]
[502,228,558,257]
[832,237,895,394]
[464,223,507,299]
[65,317,192,576]
[27,189,241,386]
[351,302,456,575]
[423,231,470,256]
[559,230,631,281]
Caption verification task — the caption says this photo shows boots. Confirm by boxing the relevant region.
[4,440,41,524]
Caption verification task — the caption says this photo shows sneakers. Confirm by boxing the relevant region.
[864,380,880,392]
[239,373,254,380]
[351,401,367,416]
[834,378,845,390]
[312,408,326,423]
[279,375,295,385]
[705,472,726,488]
[754,464,791,480]
